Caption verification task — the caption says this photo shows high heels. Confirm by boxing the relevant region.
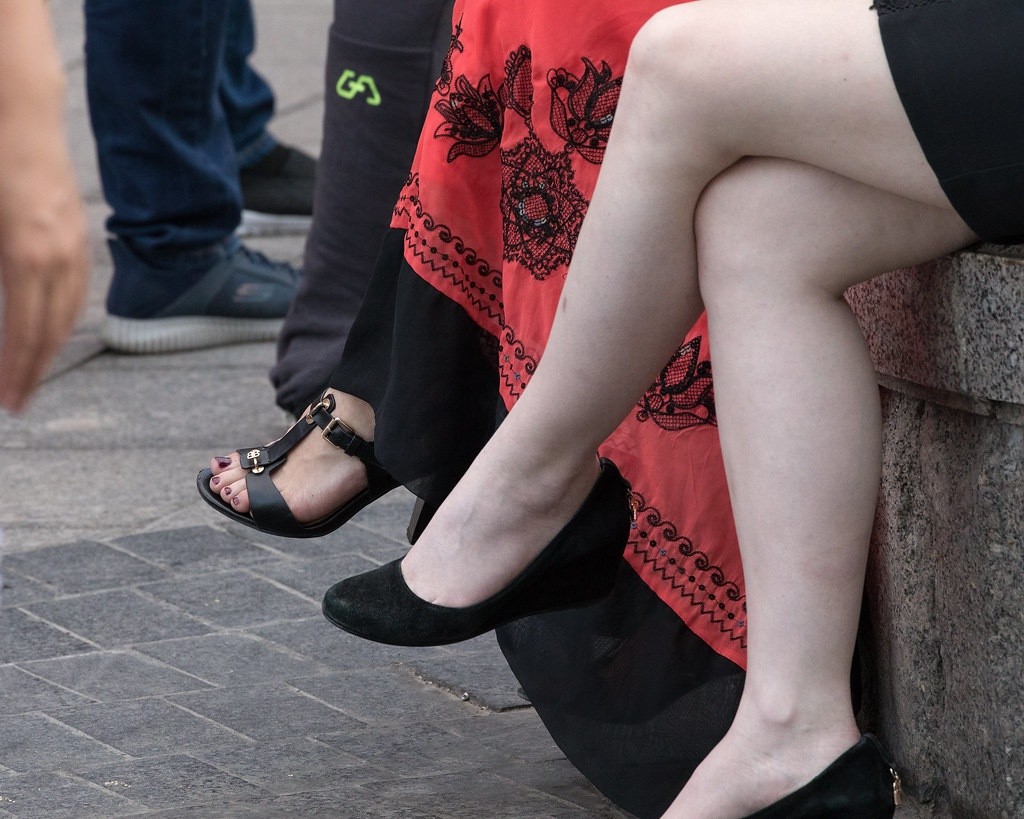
[196,387,441,546]
[322,458,645,647]
[741,732,905,819]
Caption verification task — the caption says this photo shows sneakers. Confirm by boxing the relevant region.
[231,145,318,238]
[102,237,305,354]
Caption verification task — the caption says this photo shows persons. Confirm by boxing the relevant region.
[269,0,454,416]
[322,0,1023,819]
[85,0,321,351]
[198,0,749,819]
[0,0,85,410]
